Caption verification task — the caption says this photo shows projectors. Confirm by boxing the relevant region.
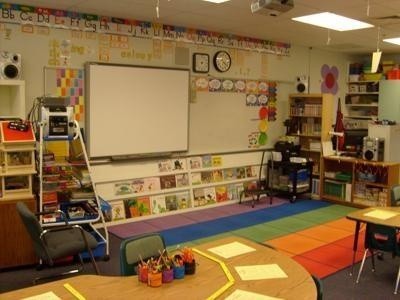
[250,0,294,18]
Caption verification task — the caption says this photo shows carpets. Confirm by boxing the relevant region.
[107,195,400,281]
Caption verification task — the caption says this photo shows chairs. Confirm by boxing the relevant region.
[312,274,323,299]
[355,224,400,295]
[17,202,102,284]
[119,233,165,276]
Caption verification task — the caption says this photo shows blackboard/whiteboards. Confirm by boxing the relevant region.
[83,61,191,164]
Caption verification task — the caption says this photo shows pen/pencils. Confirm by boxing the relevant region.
[139,250,196,274]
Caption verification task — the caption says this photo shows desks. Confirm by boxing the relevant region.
[0,233,318,299]
[345,206,400,278]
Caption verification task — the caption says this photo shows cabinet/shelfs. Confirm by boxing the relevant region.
[323,155,400,210]
[289,93,335,201]
[344,81,379,120]
[35,123,110,262]
[368,124,400,164]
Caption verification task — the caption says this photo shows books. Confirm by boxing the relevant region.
[283,96,322,198]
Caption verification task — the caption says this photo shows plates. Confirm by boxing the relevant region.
[259,134,267,145]
[260,107,267,119]
[259,120,268,133]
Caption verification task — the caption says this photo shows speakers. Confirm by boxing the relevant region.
[0,52,22,82]
[295,75,308,94]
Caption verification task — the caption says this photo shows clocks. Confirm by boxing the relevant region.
[192,53,209,73]
[214,51,231,72]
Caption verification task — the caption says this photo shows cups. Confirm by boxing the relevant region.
[173,265,185,279]
[138,267,147,282]
[185,260,195,273]
[147,272,162,287]
[162,269,174,282]
